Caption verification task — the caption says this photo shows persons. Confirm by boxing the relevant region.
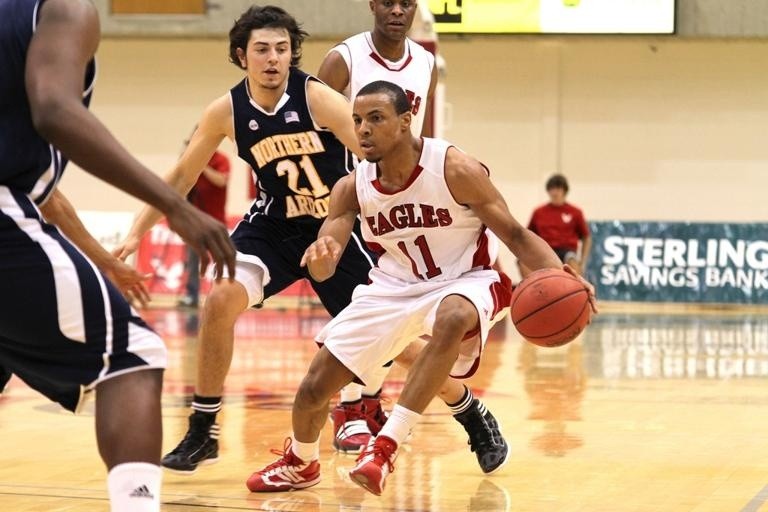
[246,80,599,497]
[517,172,593,280]
[175,124,229,311]
[315,1,441,457]
[101,5,513,478]
[0,0,238,512]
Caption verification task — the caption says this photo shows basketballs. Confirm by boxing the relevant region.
[509,267,593,349]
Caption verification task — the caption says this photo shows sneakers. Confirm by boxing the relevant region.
[331,388,388,453]
[349,434,400,496]
[246,491,321,512]
[332,452,367,501]
[246,446,321,492]
[467,480,510,512]
[161,413,220,475]
[454,399,508,474]
